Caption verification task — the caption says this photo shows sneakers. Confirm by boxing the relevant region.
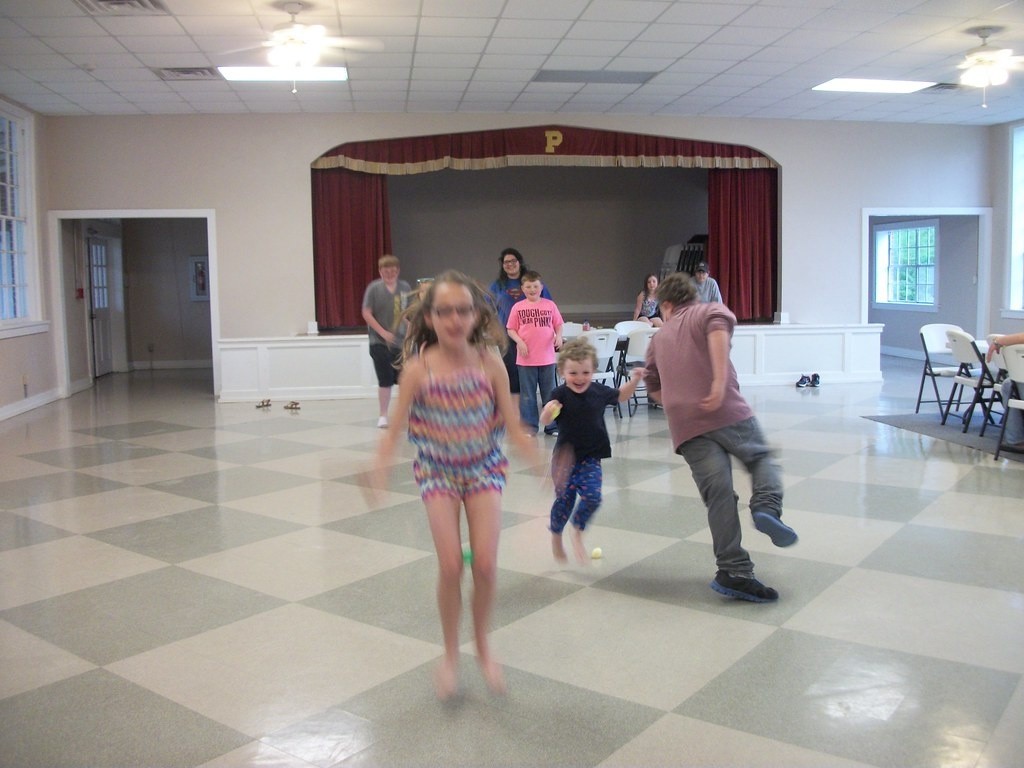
[796,375,811,387]
[752,506,798,549]
[810,374,820,386]
[710,571,779,603]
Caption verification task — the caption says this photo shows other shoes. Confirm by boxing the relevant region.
[378,416,388,428]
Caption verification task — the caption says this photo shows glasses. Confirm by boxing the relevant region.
[503,259,519,265]
[417,277,435,286]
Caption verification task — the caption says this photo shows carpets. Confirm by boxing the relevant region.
[861,410,1024,464]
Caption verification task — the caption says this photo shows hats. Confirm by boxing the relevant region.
[694,261,710,272]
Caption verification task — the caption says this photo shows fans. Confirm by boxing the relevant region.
[937,30,1024,91]
[216,1,380,62]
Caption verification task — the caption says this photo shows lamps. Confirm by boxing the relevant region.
[268,44,324,94]
[962,65,1009,108]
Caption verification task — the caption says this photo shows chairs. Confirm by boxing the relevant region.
[914,323,1024,460]
[660,234,708,282]
[555,322,661,419]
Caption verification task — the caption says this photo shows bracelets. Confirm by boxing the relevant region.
[993,337,1003,348]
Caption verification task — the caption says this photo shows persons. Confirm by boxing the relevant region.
[362,255,415,426]
[485,248,552,393]
[987,333,1024,362]
[506,271,564,438]
[634,272,666,327]
[691,262,722,303]
[643,274,798,602]
[364,271,542,701]
[540,342,643,562]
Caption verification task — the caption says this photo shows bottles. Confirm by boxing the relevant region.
[583,319,590,331]
